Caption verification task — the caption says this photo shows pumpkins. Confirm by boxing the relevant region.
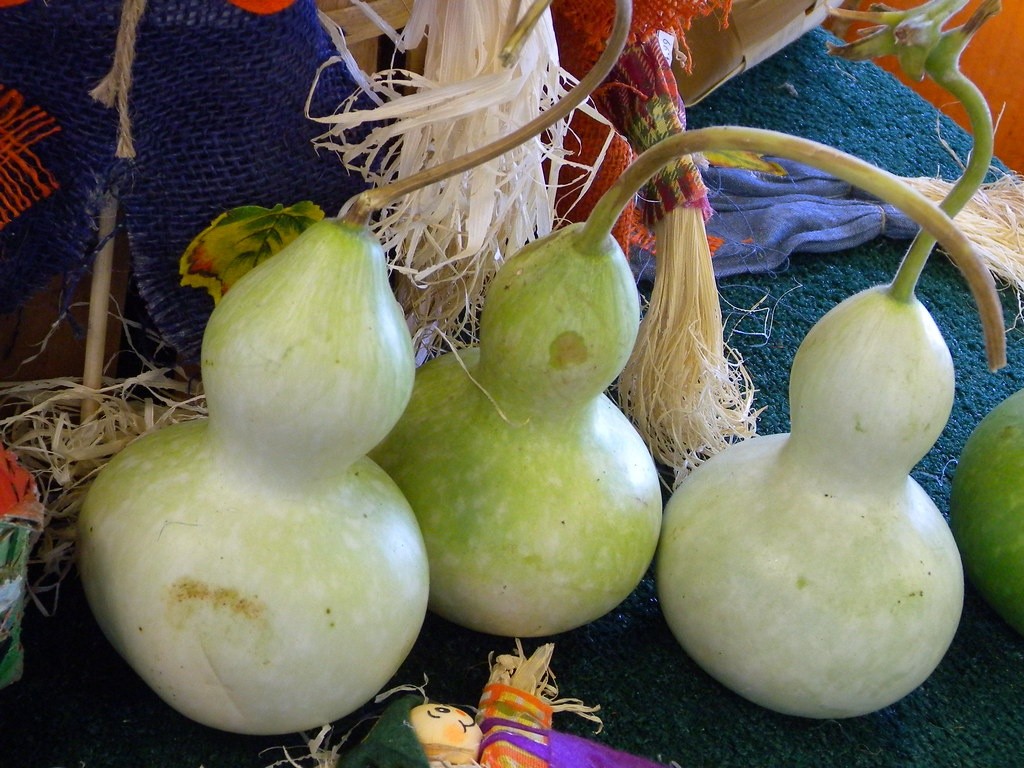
[837,0,1024,179]
